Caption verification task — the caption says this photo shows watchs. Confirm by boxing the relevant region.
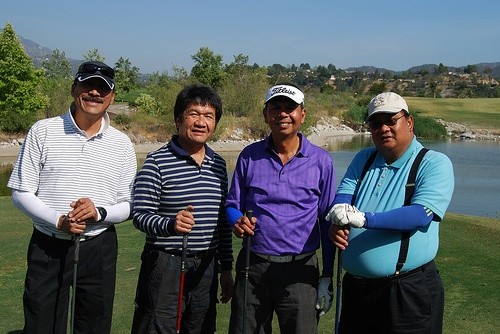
[96,206,107,223]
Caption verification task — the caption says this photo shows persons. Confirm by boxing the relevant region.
[6,61,137,334]
[226,82,336,334]
[130,85,234,334]
[325,93,455,334]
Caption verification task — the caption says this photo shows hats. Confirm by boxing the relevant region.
[74,61,115,90]
[263,85,304,107]
[365,92,409,124]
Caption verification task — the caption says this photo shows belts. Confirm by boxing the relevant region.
[148,244,217,258]
[256,251,315,263]
[34,224,93,241]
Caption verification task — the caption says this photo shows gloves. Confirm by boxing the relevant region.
[325,203,365,228]
[316,277,333,316]
[220,269,235,304]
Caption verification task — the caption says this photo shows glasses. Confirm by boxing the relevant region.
[369,114,405,131]
